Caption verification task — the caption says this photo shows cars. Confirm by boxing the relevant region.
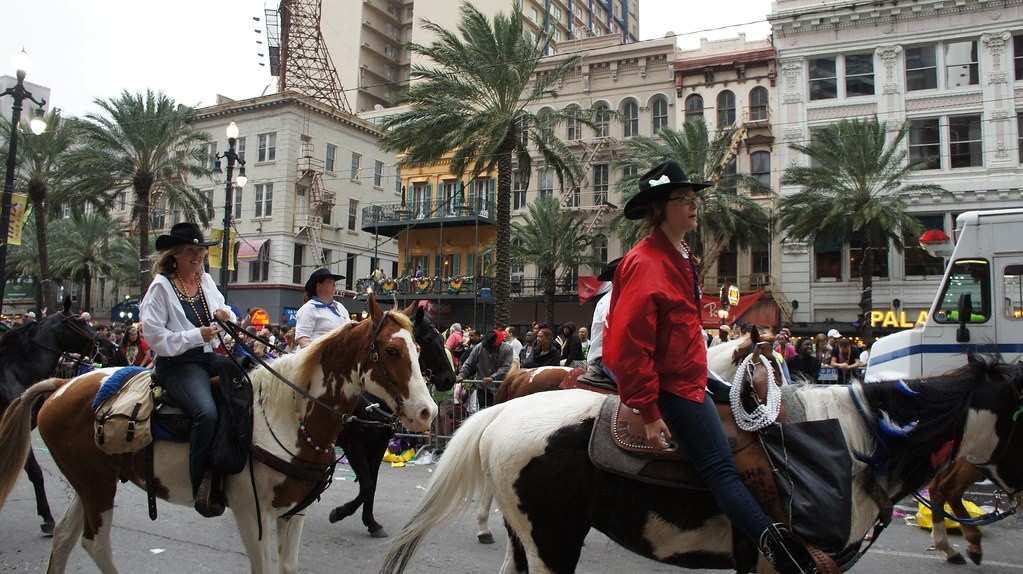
[110,295,143,323]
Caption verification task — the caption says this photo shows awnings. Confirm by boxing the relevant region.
[238,238,270,262]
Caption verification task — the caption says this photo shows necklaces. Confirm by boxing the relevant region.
[170,276,200,302]
[729,353,782,431]
[176,271,211,327]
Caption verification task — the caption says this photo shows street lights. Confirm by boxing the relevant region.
[211,122,247,305]
[0,69,47,313]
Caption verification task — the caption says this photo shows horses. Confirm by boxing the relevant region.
[0,294,129,535]
[474,325,787,544]
[388,349,1023,574]
[0,292,438,574]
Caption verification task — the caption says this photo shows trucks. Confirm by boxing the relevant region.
[865,209,1023,382]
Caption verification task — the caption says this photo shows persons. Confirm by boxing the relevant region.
[211,321,295,374]
[139,223,237,519]
[429,322,591,455]
[295,268,352,351]
[706,321,877,384]
[0,309,154,472]
[602,160,861,574]
[370,266,422,295]
[587,257,623,384]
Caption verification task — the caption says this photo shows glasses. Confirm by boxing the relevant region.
[666,193,699,204]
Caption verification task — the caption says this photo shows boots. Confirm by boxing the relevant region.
[760,521,816,574]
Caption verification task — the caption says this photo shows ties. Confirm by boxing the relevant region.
[312,295,340,316]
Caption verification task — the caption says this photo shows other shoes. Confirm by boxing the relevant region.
[196,491,224,516]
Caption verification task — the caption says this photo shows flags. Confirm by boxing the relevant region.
[578,275,612,305]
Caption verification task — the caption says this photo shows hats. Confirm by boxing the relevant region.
[304,268,346,293]
[720,325,730,334]
[483,330,503,349]
[828,329,842,339]
[155,222,222,250]
[624,159,712,220]
[597,256,625,282]
